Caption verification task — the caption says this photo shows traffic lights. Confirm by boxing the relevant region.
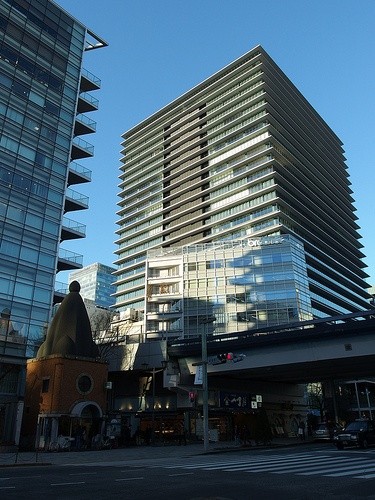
[216,352,234,360]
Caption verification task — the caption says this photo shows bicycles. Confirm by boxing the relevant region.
[48,437,73,453]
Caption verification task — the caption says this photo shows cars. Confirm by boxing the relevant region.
[336,418,375,450]
[311,422,342,444]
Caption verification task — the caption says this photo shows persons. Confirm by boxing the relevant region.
[122,422,143,447]
[262,419,272,446]
[88,427,98,449]
[234,423,252,446]
[177,422,187,446]
[75,425,84,451]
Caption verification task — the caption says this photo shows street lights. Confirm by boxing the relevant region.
[192,317,217,453]
